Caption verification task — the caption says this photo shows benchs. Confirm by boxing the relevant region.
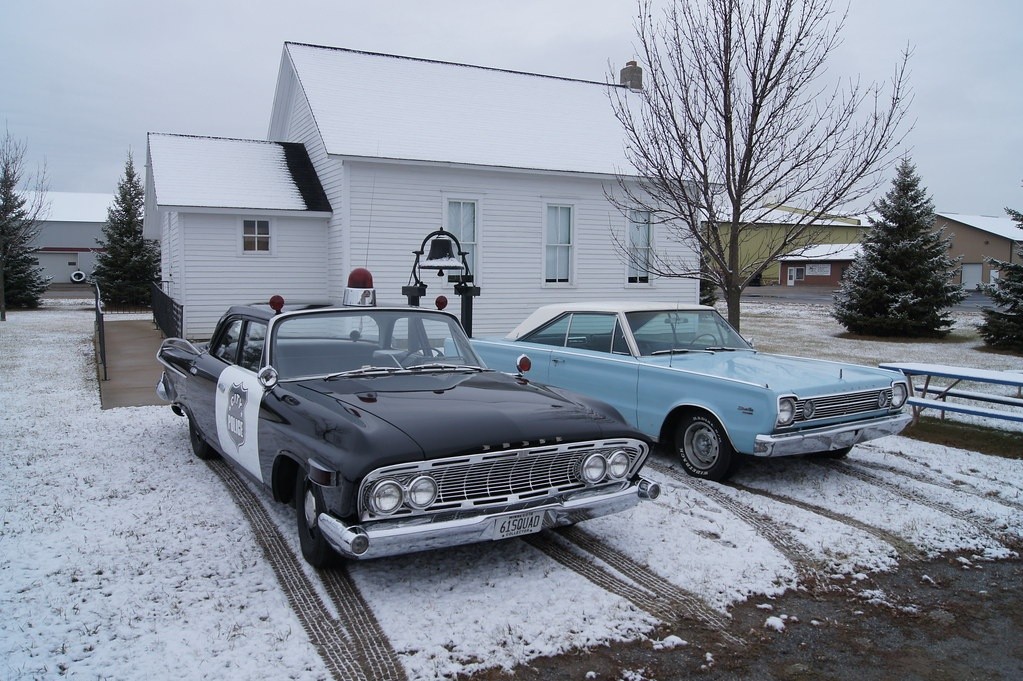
[906,384,1023,422]
[227,337,384,375]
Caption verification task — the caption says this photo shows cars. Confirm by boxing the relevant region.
[441,301,915,482]
[155,267,662,567]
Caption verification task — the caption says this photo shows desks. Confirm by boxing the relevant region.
[878,363,1023,427]
